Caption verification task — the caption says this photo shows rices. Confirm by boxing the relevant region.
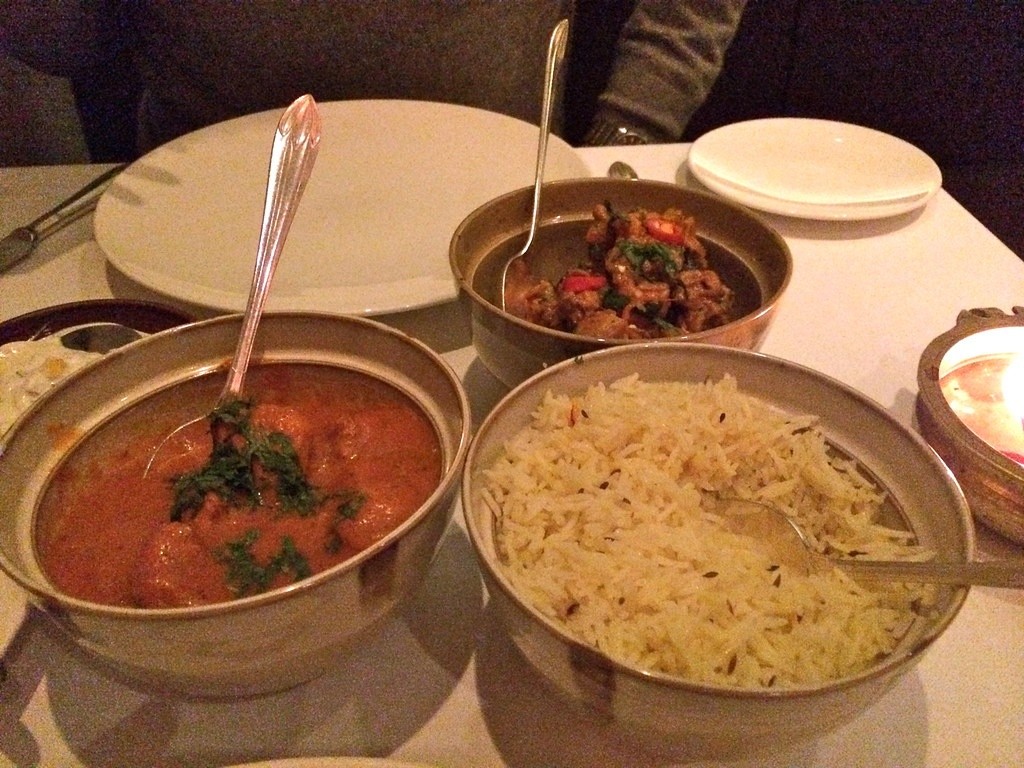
[479,372,938,689]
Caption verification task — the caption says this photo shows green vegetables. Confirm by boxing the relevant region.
[167,394,364,597]
[576,196,687,332]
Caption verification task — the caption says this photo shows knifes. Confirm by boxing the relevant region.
[0,165,133,276]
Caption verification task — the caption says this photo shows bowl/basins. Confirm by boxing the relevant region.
[0,176,1024,767]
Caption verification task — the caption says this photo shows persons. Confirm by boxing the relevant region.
[0,0,747,148]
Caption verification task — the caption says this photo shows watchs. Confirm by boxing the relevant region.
[586,120,648,147]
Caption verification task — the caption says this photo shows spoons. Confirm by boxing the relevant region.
[709,496,1024,590]
[142,92,320,483]
[502,18,570,316]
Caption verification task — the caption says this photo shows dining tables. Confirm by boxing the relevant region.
[1,142,1024,767]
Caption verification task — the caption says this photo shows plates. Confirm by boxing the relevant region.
[93,98,590,316]
[684,115,941,223]
[1,298,196,446]
